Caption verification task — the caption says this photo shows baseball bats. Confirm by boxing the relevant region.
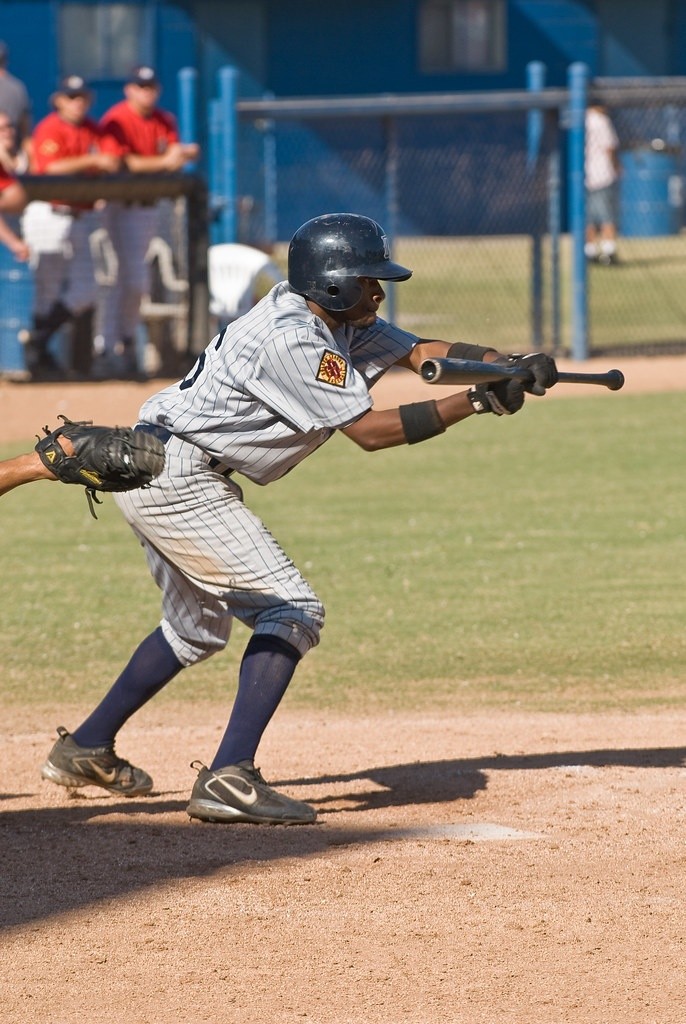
[419,357,625,392]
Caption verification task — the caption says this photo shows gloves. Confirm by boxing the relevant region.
[492,352,558,396]
[467,382,527,416]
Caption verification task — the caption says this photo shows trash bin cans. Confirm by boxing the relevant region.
[616,141,685,237]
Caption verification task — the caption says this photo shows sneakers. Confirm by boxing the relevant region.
[42,726,153,797]
[186,760,317,826]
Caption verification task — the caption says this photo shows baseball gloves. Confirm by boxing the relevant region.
[34,414,167,520]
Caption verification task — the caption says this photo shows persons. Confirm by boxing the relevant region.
[0,42,199,383]
[0,434,74,497]
[585,103,624,265]
[41,212,559,824]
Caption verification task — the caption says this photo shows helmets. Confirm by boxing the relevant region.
[288,213,413,312]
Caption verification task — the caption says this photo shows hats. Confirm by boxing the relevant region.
[59,73,88,97]
[125,64,161,87]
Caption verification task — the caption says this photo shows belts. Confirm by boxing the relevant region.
[52,206,80,217]
[135,424,237,477]
[125,197,174,208]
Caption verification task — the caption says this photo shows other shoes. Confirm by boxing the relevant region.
[123,366,149,382]
[76,360,109,380]
[24,343,60,381]
